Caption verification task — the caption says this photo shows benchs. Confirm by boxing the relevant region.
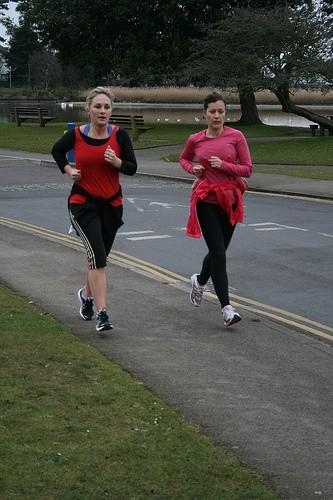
[109,113,154,139]
[9,106,58,127]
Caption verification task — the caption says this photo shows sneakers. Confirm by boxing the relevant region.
[220,303,242,326]
[190,273,207,307]
[78,288,94,322]
[95,306,114,332]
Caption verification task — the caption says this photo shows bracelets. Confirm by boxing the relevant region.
[120,160,121,167]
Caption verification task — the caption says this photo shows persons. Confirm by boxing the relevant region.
[178,91,253,326]
[50,85,138,332]
